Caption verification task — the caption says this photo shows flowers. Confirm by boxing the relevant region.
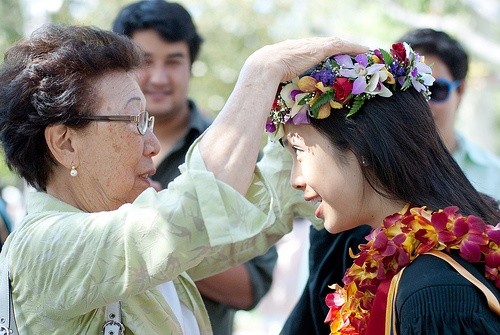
[265,40,436,146]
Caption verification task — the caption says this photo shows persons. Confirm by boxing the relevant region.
[114,0,280,335]
[394,28,500,206]
[268,49,500,335]
[0,24,370,335]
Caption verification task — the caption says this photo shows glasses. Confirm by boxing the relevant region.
[427,80,460,101]
[72,111,154,134]
